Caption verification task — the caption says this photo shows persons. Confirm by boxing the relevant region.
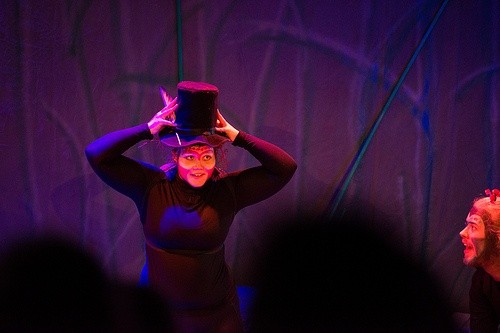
[83,80,301,330]
[458,189,500,333]
[0,193,458,333]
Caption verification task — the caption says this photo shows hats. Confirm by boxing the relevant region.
[158,80,231,148]
